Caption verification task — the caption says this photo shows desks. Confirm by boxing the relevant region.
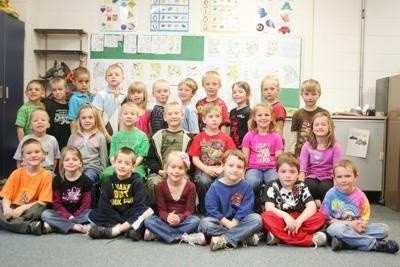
[283,112,387,207]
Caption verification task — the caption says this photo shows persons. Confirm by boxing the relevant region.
[85,145,155,241]
[40,144,96,234]
[258,150,329,249]
[318,158,400,253]
[198,147,263,251]
[289,79,340,204]
[0,137,54,235]
[142,149,207,246]
[12,63,287,214]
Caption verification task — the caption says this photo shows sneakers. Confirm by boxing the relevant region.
[266,231,280,246]
[144,228,160,241]
[210,235,229,251]
[124,226,143,241]
[311,231,327,249]
[178,232,207,248]
[331,235,349,251]
[29,221,53,235]
[243,233,259,248]
[375,238,399,253]
[88,224,112,239]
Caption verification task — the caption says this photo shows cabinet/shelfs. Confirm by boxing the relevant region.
[32,28,89,99]
[0,9,26,185]
